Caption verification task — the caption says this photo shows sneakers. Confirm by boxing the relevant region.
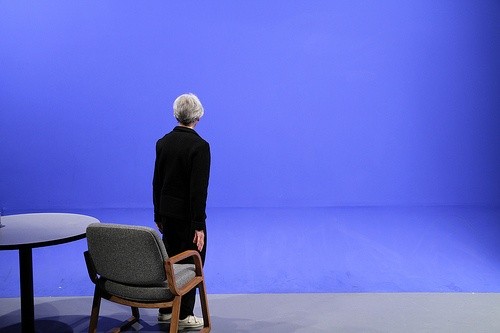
[157,313,172,321]
[178,315,203,330]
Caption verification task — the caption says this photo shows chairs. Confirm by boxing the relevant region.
[83,222,212,333]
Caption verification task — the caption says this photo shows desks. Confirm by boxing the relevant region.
[0,213,107,333]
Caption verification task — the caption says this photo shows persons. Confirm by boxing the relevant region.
[152,93,211,330]
